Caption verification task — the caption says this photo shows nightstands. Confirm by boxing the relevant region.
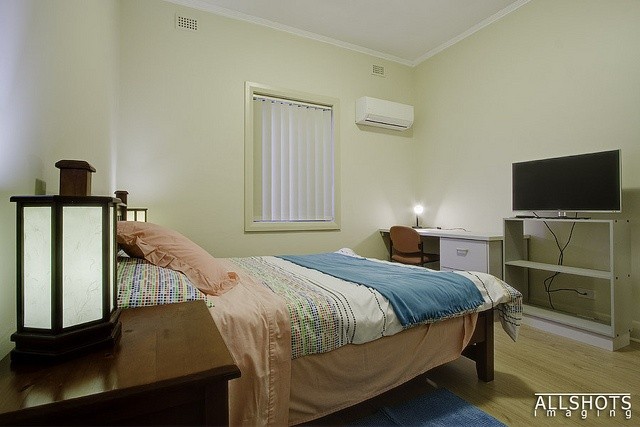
[0,298,240,425]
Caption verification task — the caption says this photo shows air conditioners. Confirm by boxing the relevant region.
[355,96,414,131]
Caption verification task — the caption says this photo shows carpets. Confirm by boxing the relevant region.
[349,390,508,427]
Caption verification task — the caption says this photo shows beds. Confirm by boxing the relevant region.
[54,158,518,427]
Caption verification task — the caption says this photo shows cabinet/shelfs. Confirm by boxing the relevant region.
[501,218,632,351]
[440,237,489,274]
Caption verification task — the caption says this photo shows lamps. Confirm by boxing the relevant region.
[9,195,124,361]
[126,207,148,222]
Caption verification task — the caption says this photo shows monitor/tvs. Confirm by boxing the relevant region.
[511,148,622,220]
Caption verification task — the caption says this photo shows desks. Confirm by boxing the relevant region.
[378,227,529,299]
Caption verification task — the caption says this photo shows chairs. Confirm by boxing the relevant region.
[389,225,440,266]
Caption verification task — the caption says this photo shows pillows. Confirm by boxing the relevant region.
[115,256,214,309]
[116,222,236,295]
